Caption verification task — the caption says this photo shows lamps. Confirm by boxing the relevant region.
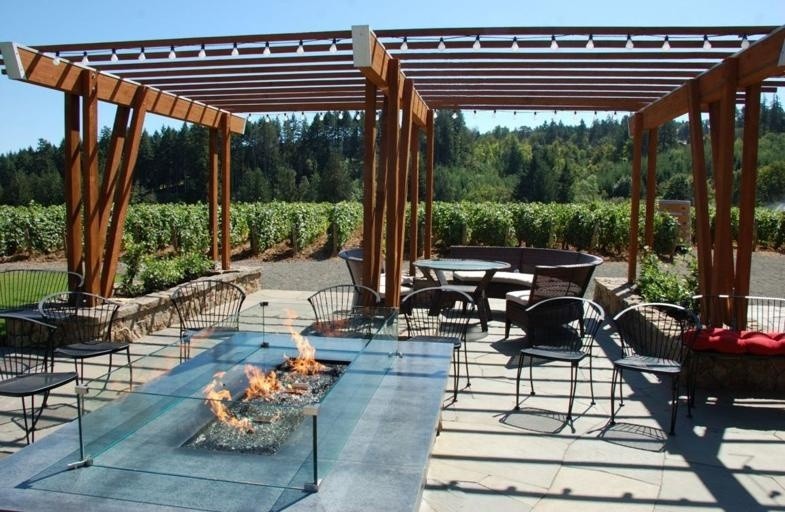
[394,34,756,55]
[49,39,346,68]
[432,108,621,120]
[245,110,380,122]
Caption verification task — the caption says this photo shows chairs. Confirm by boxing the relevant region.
[0,269,133,472]
[169,279,246,365]
[339,247,414,317]
[308,285,476,403]
[515,297,701,437]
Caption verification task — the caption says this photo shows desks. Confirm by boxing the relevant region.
[411,258,512,332]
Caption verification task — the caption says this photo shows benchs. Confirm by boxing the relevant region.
[683,294,785,409]
[449,245,604,340]
